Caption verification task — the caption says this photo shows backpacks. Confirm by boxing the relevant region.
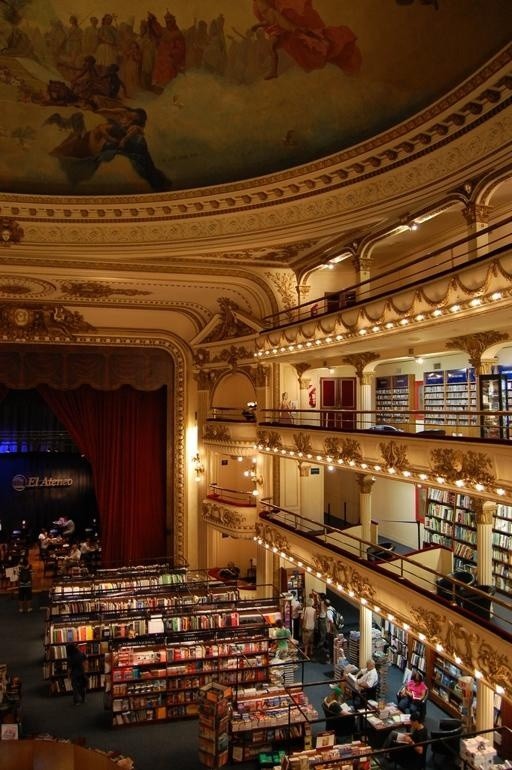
[332,609,345,630]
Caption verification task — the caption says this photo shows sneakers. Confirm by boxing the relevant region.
[19,607,33,612]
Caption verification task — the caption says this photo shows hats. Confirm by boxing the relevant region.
[22,558,29,566]
[333,687,343,698]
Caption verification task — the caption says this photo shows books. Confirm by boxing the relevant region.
[374,614,476,720]
[40,564,240,693]
[421,485,511,595]
[1,664,22,707]
[40,734,134,769]
[201,685,319,769]
[111,626,270,727]
[282,740,373,769]
[269,657,297,687]
[377,379,512,427]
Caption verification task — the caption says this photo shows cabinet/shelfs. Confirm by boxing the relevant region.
[374,380,512,439]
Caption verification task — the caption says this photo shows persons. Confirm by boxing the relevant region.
[42,515,102,565]
[382,672,427,764]
[321,659,378,734]
[293,592,338,664]
[66,643,90,706]
[274,619,291,658]
[16,559,32,612]
